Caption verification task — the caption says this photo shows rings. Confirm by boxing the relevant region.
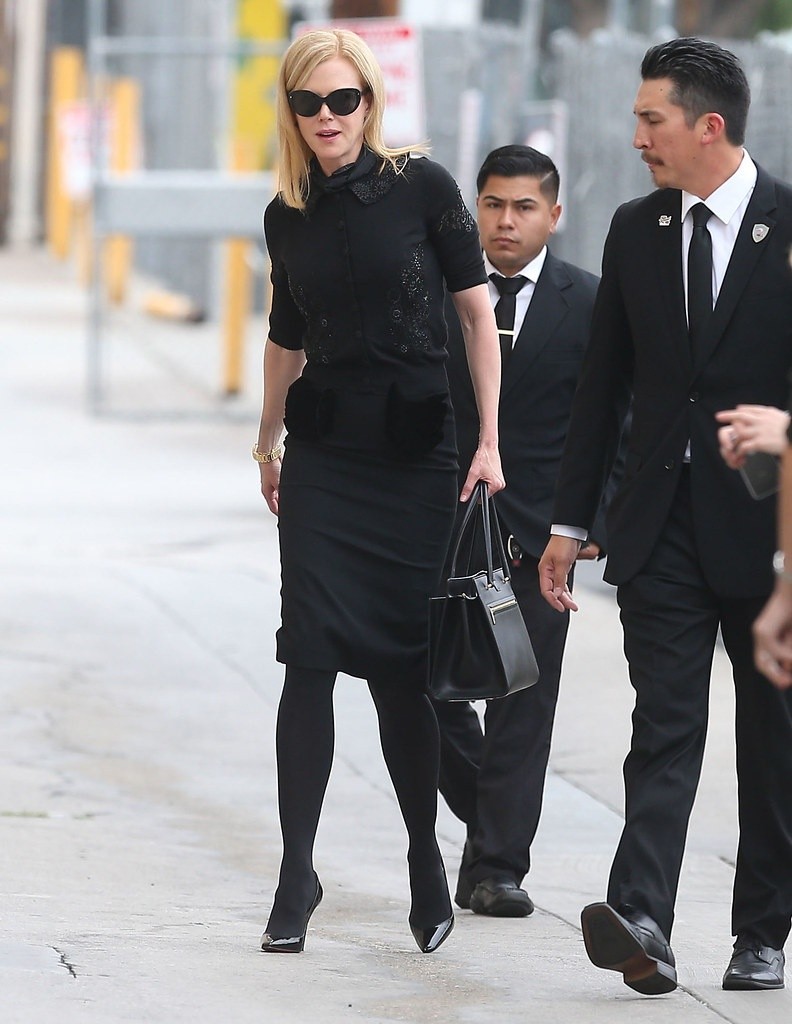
[729,432,740,444]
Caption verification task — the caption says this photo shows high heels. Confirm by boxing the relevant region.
[407,848,455,953]
[261,870,323,952]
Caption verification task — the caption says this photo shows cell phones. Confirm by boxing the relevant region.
[732,436,781,500]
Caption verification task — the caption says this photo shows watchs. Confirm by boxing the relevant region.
[253,443,284,463]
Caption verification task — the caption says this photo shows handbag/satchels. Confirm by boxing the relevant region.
[425,481,539,703]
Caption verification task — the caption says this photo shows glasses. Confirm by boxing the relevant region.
[286,88,370,117]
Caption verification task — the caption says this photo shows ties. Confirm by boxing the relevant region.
[688,202,713,360]
[489,274,529,368]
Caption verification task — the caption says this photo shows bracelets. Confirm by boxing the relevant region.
[771,547,792,584]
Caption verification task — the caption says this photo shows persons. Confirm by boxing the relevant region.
[253,27,509,954]
[714,402,792,686]
[538,39,792,991]
[435,145,610,919]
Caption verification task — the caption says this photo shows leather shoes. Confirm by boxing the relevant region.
[580,901,679,996]
[722,945,785,988]
[469,876,533,916]
